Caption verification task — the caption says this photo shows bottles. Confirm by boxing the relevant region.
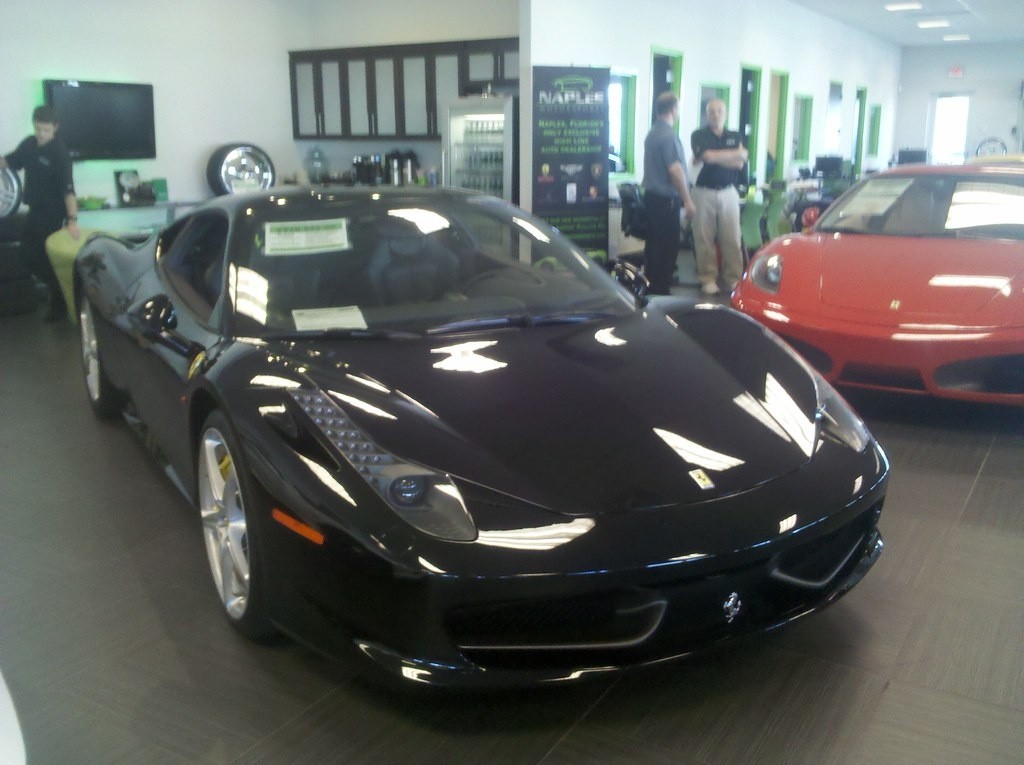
[312,152,323,183]
[461,120,503,197]
[370,155,384,184]
[351,156,367,182]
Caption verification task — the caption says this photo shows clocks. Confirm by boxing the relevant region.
[114,169,141,208]
[207,142,278,197]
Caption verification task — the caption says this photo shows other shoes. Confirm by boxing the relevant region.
[729,280,738,292]
[44,296,67,323]
[701,282,720,294]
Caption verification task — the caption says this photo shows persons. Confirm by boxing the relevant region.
[0,104,80,320]
[690,95,749,294]
[639,91,692,297]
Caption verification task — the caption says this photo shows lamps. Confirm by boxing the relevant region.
[882,3,972,42]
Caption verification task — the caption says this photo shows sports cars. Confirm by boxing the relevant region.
[729,164,1024,417]
[72,184,892,715]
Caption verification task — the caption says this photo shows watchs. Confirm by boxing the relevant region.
[64,216,77,221]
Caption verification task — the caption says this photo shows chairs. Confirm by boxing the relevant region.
[242,244,325,321]
[880,189,954,237]
[382,233,464,309]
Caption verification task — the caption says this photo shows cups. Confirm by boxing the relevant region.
[403,159,412,185]
[389,159,399,186]
